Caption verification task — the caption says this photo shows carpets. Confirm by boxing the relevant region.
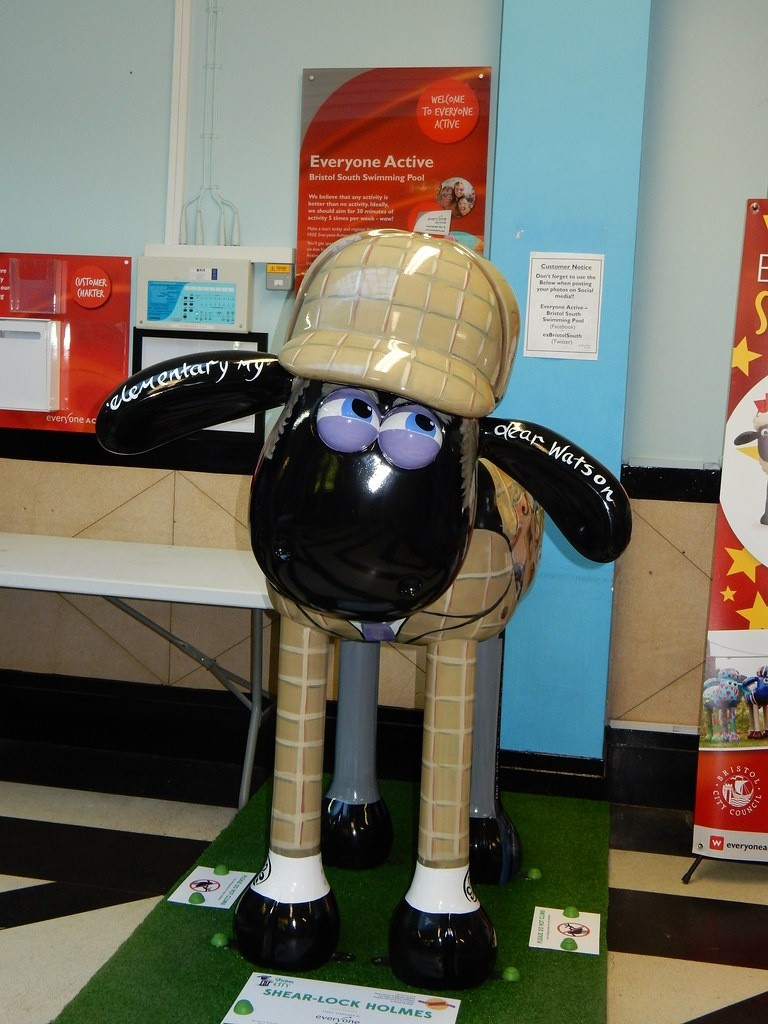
[49,767,610,1024]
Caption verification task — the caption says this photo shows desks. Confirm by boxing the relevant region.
[0,531,276,813]
